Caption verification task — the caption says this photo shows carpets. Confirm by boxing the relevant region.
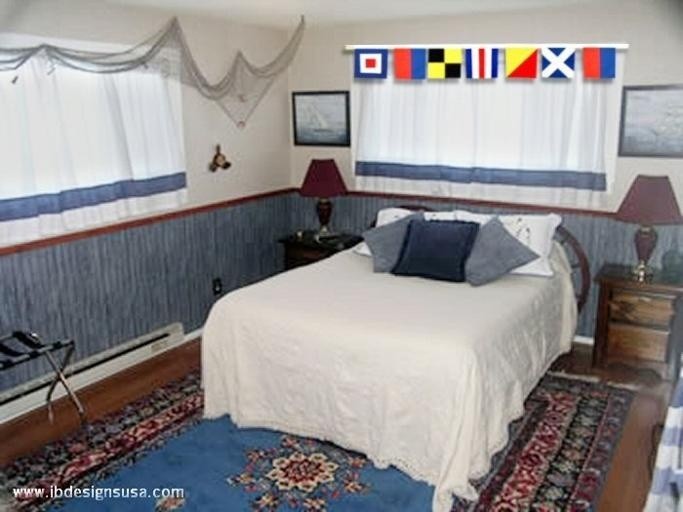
[0,366,644,511]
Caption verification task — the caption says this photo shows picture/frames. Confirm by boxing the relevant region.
[617,84,683,158]
[290,90,351,147]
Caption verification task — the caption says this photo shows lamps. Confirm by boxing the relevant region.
[613,175,683,280]
[299,158,348,244]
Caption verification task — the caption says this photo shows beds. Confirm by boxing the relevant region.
[198,206,590,512]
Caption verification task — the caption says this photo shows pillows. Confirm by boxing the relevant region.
[352,207,563,287]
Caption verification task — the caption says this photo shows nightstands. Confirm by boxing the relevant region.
[592,260,683,380]
[276,230,364,271]
